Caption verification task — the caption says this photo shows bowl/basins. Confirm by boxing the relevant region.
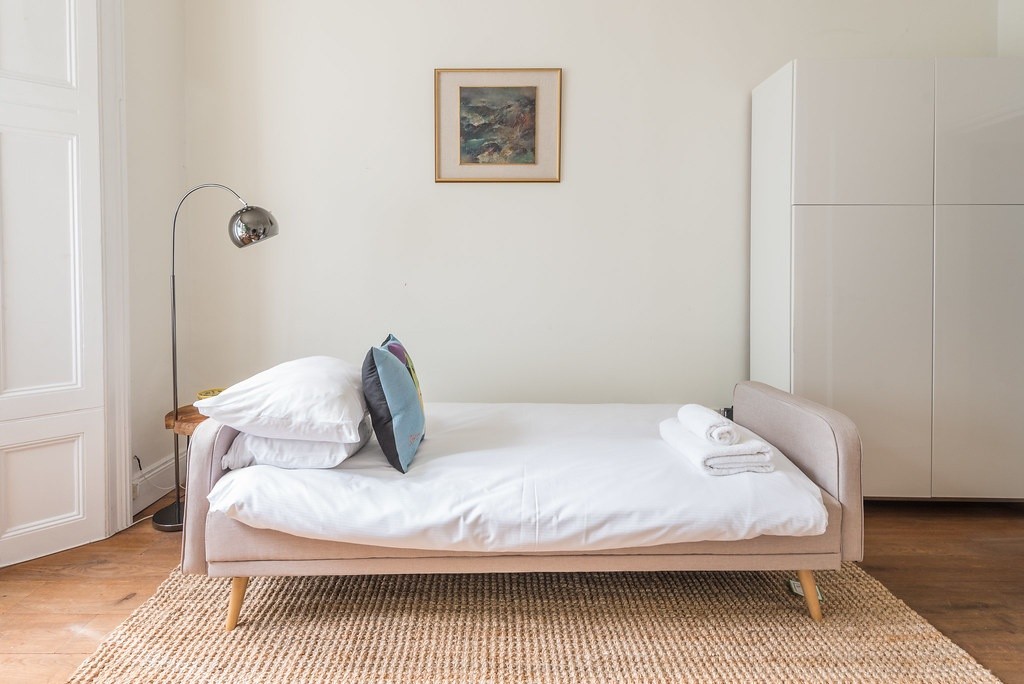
[197,388,224,400]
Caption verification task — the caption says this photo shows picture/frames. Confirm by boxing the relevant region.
[434,68,562,182]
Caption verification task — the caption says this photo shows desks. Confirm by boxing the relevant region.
[164,405,210,453]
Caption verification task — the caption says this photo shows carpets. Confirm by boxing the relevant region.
[67,562,1011,684]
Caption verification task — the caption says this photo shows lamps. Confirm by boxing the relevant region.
[153,183,279,532]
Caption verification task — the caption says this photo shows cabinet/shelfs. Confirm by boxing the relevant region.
[749,57,1023,501]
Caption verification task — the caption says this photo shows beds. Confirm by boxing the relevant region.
[180,381,865,631]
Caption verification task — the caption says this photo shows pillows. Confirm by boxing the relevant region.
[221,416,373,470]
[193,356,370,444]
[361,333,427,474]
